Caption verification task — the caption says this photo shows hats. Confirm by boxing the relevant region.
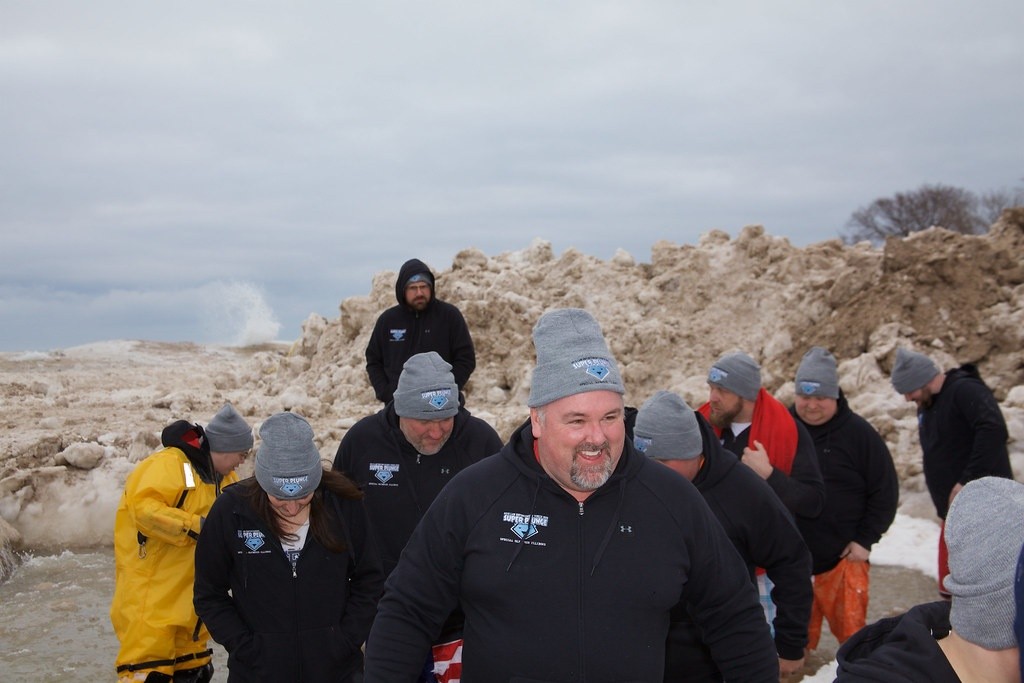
[204,404,253,452]
[527,308,627,408]
[943,476,1024,648]
[706,352,761,402]
[890,343,940,394]
[254,413,323,499]
[633,391,704,459]
[404,275,433,290]
[795,345,839,400]
[392,353,459,420]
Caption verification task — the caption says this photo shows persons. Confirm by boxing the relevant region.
[833,476,1024,683]
[632,391,815,683]
[366,259,476,405]
[109,407,254,683]
[891,347,1013,601]
[364,307,781,683]
[331,352,504,683]
[192,413,379,683]
[696,353,823,640]
[788,347,900,660]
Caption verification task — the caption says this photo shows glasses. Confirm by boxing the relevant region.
[238,453,250,460]
